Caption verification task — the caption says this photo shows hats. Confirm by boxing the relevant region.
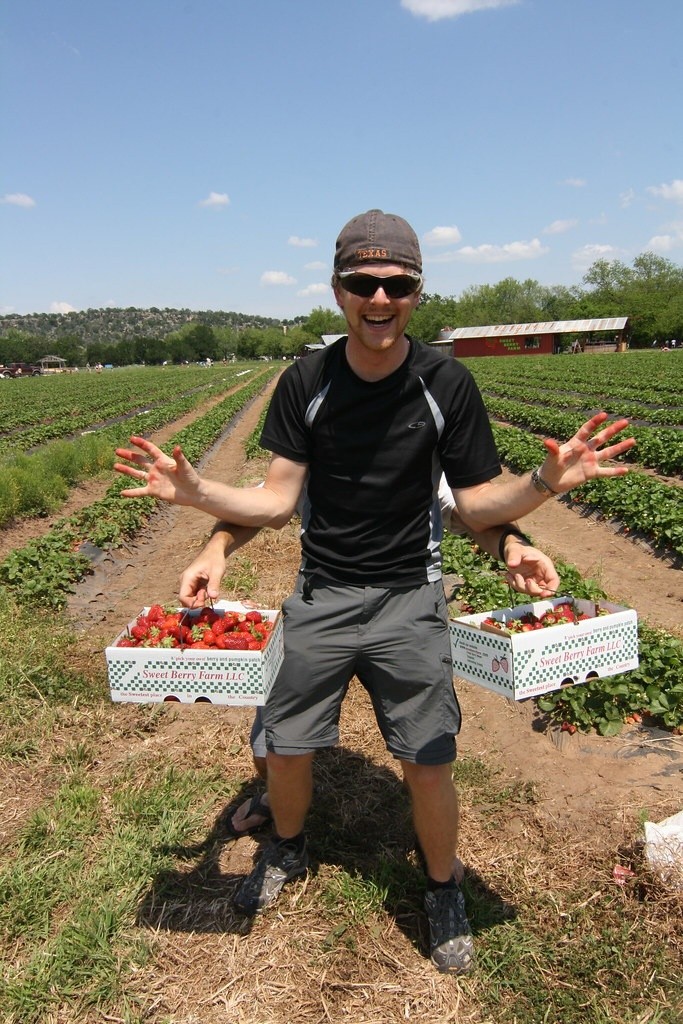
[331,207,423,273]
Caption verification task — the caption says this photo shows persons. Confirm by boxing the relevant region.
[86,362,102,374]
[197,357,214,367]
[112,210,637,974]
[177,471,559,839]
[661,339,677,351]
[283,356,286,363]
[223,360,226,366]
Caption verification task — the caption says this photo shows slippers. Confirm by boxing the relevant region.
[227,791,275,835]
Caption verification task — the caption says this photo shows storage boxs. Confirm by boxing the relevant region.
[448,595,640,702]
[105,598,286,707]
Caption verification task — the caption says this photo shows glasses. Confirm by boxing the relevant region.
[337,272,422,299]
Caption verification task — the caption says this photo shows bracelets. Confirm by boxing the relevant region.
[499,529,534,563]
[531,469,558,499]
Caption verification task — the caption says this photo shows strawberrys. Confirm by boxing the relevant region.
[481,601,595,636]
[461,605,475,614]
[561,721,576,734]
[626,709,650,724]
[116,603,274,651]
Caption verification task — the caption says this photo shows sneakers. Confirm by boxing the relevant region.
[424,877,478,976]
[230,832,310,917]
